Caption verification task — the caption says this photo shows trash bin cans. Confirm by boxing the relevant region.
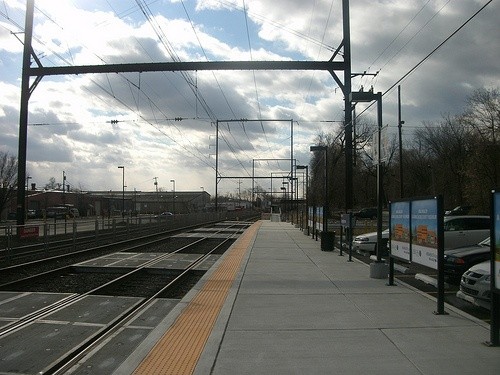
[320,230,336,251]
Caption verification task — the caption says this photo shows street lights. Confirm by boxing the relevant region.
[118,166,124,224]
[170,180,175,221]
[296,166,310,235]
[200,186,204,213]
[25,176,33,220]
[281,178,300,228]
[349,92,389,279]
[310,146,327,232]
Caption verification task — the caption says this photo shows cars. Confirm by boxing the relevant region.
[352,207,378,220]
[456,259,500,316]
[352,228,389,256]
[445,204,468,216]
[443,215,491,250]
[154,212,172,221]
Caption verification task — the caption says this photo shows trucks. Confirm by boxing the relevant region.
[45,203,79,219]
